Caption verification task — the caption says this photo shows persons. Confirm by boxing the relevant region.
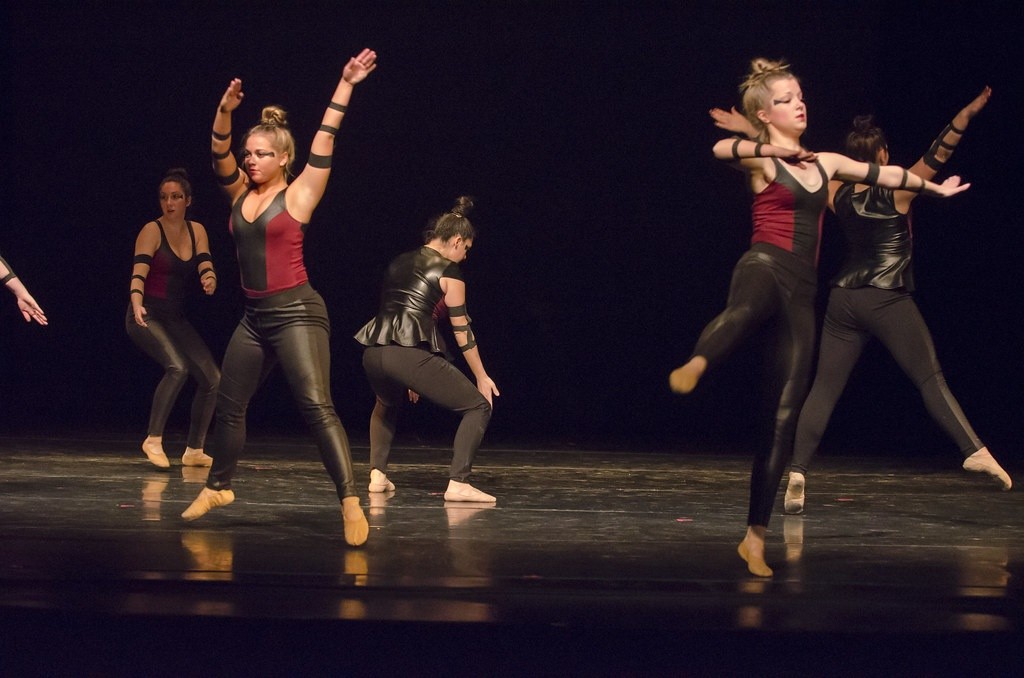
[0,256,48,325]
[124,169,221,467]
[353,197,498,502]
[709,85,1010,516]
[669,57,971,575]
[181,48,376,544]
